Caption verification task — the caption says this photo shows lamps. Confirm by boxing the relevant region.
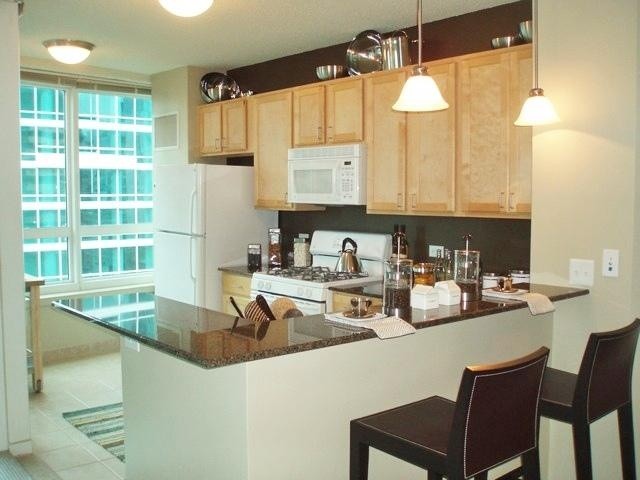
[391,64,449,113]
[41,39,95,65]
[514,84,560,126]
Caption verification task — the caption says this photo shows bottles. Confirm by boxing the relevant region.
[443,249,450,267]
[248,245,262,273]
[435,250,444,281]
[445,252,455,281]
[385,224,411,309]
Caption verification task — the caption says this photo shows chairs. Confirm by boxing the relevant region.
[541,318,640,480]
[349,346,550,480]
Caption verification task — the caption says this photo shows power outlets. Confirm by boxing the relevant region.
[602,248,619,277]
[567,258,595,286]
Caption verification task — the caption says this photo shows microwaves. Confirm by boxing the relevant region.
[287,143,365,206]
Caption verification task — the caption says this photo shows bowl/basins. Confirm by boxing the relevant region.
[315,65,347,81]
[345,29,385,77]
[492,36,516,50]
[518,20,532,43]
[199,71,241,104]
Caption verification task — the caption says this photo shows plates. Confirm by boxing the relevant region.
[342,310,376,319]
[492,286,519,294]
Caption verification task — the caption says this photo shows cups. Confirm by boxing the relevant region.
[348,295,371,316]
[497,277,513,290]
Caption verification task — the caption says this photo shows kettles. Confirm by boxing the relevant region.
[333,237,363,274]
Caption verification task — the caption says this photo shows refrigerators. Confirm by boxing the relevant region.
[151,163,279,311]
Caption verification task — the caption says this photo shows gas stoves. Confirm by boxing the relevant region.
[250,229,392,317]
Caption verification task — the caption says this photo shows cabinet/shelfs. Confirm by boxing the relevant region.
[451,44,535,219]
[52,281,589,480]
[197,98,248,159]
[359,58,456,216]
[248,91,295,210]
[291,75,363,147]
[219,261,282,319]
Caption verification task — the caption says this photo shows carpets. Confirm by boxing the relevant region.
[62,402,125,463]
